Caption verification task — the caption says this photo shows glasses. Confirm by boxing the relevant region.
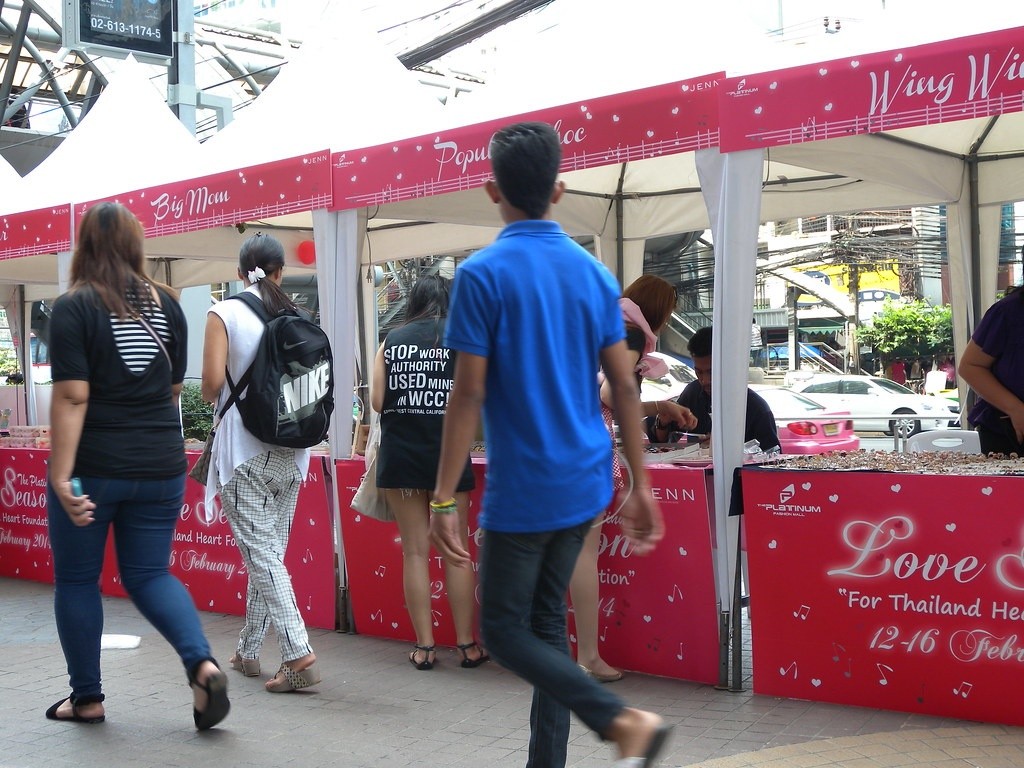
[671,285,678,309]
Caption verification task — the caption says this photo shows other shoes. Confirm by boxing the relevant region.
[577,663,624,682]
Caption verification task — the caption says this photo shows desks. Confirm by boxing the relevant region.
[0,442,336,630]
[334,455,818,686]
[729,459,1024,729]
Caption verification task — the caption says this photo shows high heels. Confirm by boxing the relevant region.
[266,662,320,693]
[231,651,260,677]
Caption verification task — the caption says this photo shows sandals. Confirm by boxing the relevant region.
[45,695,106,724]
[408,644,436,671]
[190,663,229,730]
[458,642,491,668]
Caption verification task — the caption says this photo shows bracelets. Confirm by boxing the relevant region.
[429,497,456,513]
[655,400,659,411]
[655,413,670,430]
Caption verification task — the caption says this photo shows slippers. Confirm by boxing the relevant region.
[609,721,671,768]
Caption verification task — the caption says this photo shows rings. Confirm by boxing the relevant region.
[689,412,692,416]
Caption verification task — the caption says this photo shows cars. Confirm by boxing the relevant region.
[751,342,915,396]
[789,375,962,438]
[746,385,863,455]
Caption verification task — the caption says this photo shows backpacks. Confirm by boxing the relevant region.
[223,290,335,447]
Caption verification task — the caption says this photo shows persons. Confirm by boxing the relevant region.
[199,234,320,693]
[370,275,488,669]
[750,318,763,366]
[45,200,231,732]
[957,244,1024,459]
[427,122,677,767]
[642,327,781,453]
[570,275,698,680]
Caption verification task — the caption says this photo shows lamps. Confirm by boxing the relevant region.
[237,222,249,234]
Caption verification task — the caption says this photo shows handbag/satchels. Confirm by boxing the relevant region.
[188,429,215,486]
[350,446,396,522]
[365,413,382,471]
